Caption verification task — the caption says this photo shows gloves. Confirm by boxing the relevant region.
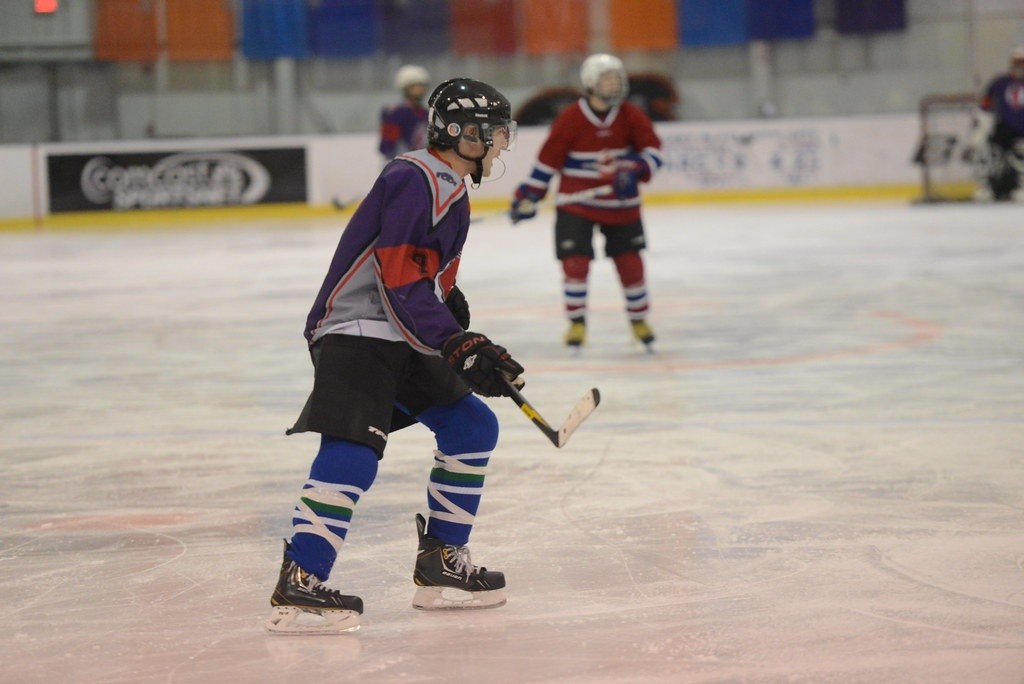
[615,169,636,199]
[441,331,526,406]
[444,279,470,331]
[512,199,534,222]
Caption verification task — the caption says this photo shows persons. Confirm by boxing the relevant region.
[377,61,431,170]
[977,46,1023,203]
[269,77,526,616]
[509,52,663,347]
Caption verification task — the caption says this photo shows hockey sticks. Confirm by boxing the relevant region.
[504,182,613,218]
[492,365,601,450]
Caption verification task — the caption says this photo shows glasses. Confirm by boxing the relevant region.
[474,124,510,143]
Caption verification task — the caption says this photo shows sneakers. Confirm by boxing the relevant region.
[411,513,507,610]
[565,323,586,353]
[631,319,656,353]
[266,538,363,635]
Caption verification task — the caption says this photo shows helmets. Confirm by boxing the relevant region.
[426,78,511,148]
[580,53,626,105]
[394,64,430,90]
